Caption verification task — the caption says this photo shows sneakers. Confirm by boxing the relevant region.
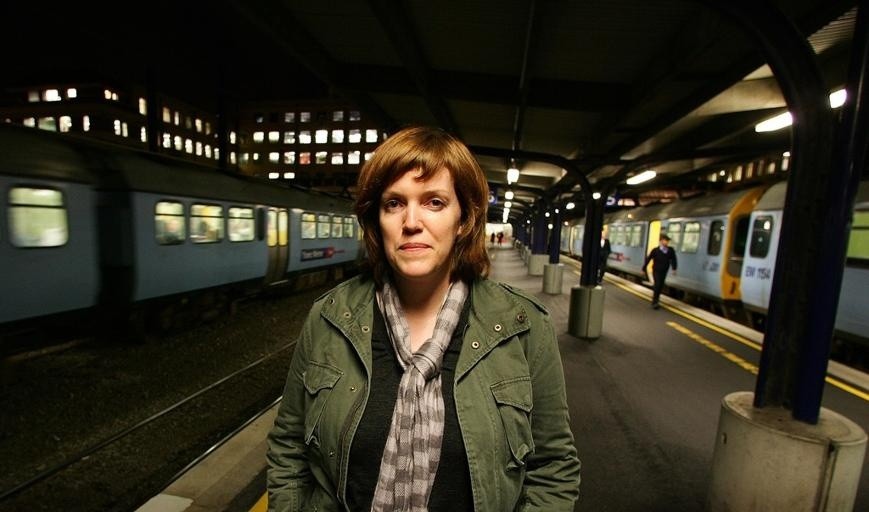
[651,302,662,311]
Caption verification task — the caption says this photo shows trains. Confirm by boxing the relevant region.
[0,121,368,358]
[559,180,869,373]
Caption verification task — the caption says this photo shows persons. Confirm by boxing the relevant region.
[496,230,504,247]
[639,233,679,311]
[263,123,583,511]
[490,232,496,247]
[599,236,614,284]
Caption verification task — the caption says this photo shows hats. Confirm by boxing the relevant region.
[659,234,671,241]
